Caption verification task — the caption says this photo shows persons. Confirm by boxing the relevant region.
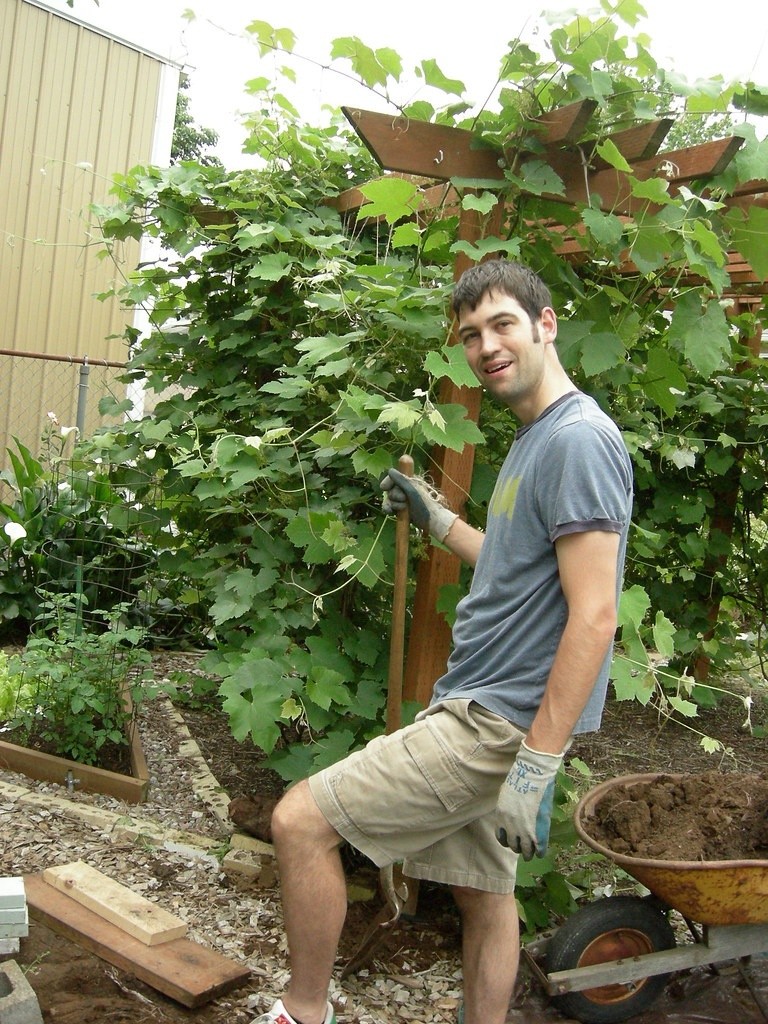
[248,261,634,1023]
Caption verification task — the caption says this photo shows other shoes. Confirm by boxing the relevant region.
[249,999,336,1024]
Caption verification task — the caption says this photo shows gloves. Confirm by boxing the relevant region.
[494,737,565,861]
[380,468,459,544]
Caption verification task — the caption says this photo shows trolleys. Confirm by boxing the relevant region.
[517,773,768,1024]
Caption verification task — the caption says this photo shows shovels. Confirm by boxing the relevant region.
[338,454,414,984]
